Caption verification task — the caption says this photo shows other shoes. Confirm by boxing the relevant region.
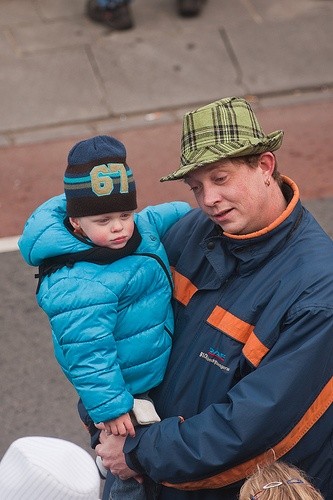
[176,0,206,17]
[86,0,134,31]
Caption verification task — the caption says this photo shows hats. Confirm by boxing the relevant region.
[61,134,138,218]
[159,96,285,183]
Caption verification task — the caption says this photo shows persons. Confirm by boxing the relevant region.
[75,97,332,500]
[0,436,100,500]
[240,461,325,500]
[18,136,192,500]
[87,0,206,30]
